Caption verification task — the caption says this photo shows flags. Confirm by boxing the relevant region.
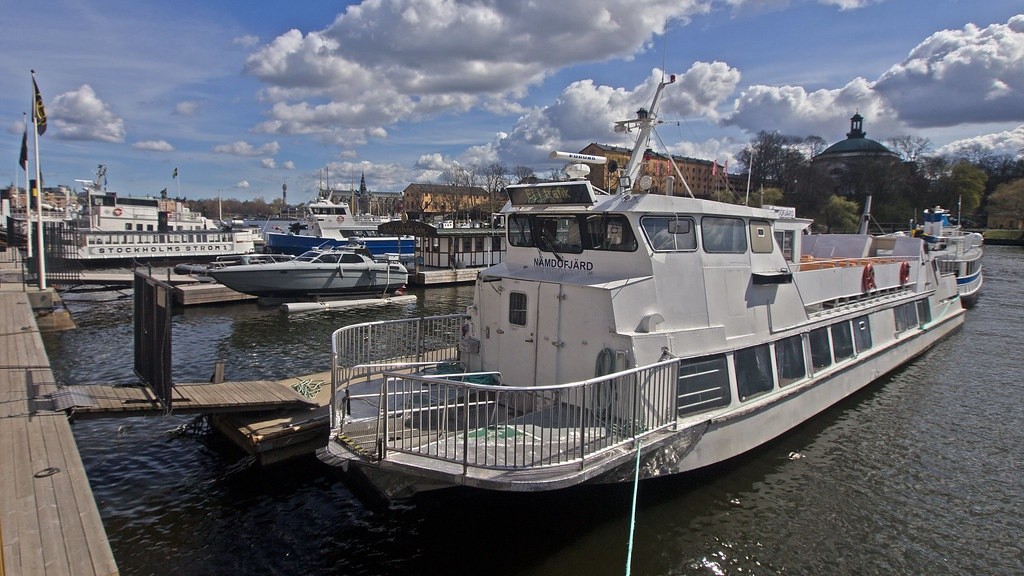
[912,226,934,243]
[172,169,178,179]
[18,132,27,169]
[31,81,47,135]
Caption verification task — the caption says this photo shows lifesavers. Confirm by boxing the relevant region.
[337,216,345,223]
[900,261,911,282]
[863,264,875,290]
[113,208,123,217]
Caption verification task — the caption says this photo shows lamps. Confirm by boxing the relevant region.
[620,177,631,191]
[641,176,656,193]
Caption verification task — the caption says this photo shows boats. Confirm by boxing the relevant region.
[315,70,970,491]
[906,196,986,301]
[206,156,509,299]
[24,162,265,285]
